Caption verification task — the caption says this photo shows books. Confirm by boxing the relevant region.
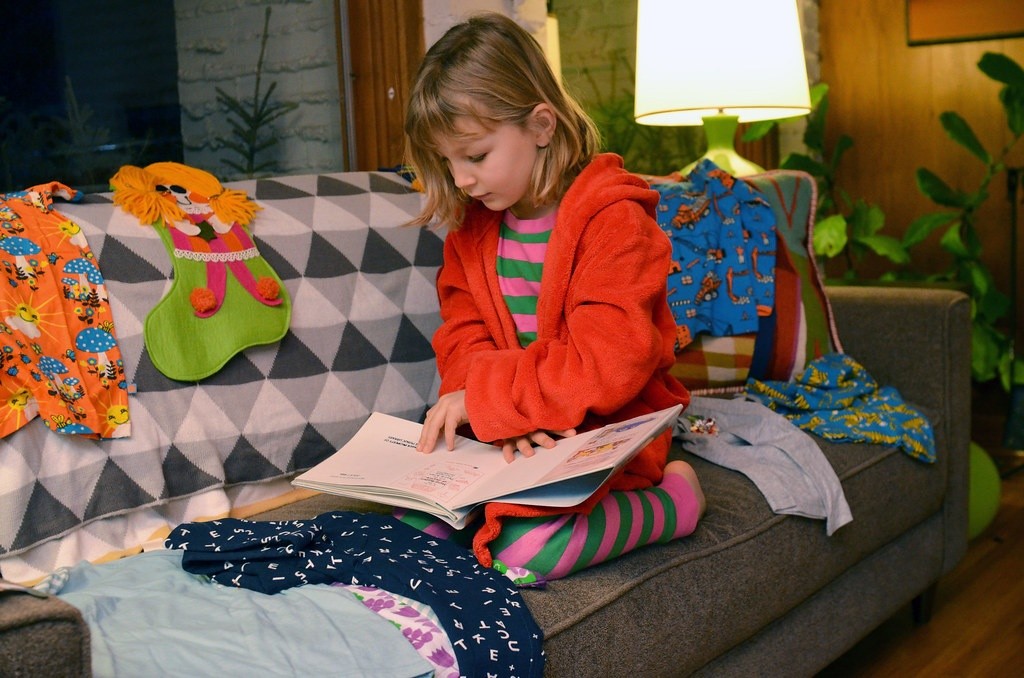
[290,404,684,530]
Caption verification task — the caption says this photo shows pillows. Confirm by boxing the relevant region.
[628,170,850,397]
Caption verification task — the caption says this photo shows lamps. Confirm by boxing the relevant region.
[633,0,810,187]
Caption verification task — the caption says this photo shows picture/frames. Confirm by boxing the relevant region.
[905,0,1024,47]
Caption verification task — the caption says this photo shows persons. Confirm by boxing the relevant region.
[389,12,705,583]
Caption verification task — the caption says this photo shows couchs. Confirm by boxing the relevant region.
[0,166,973,678]
